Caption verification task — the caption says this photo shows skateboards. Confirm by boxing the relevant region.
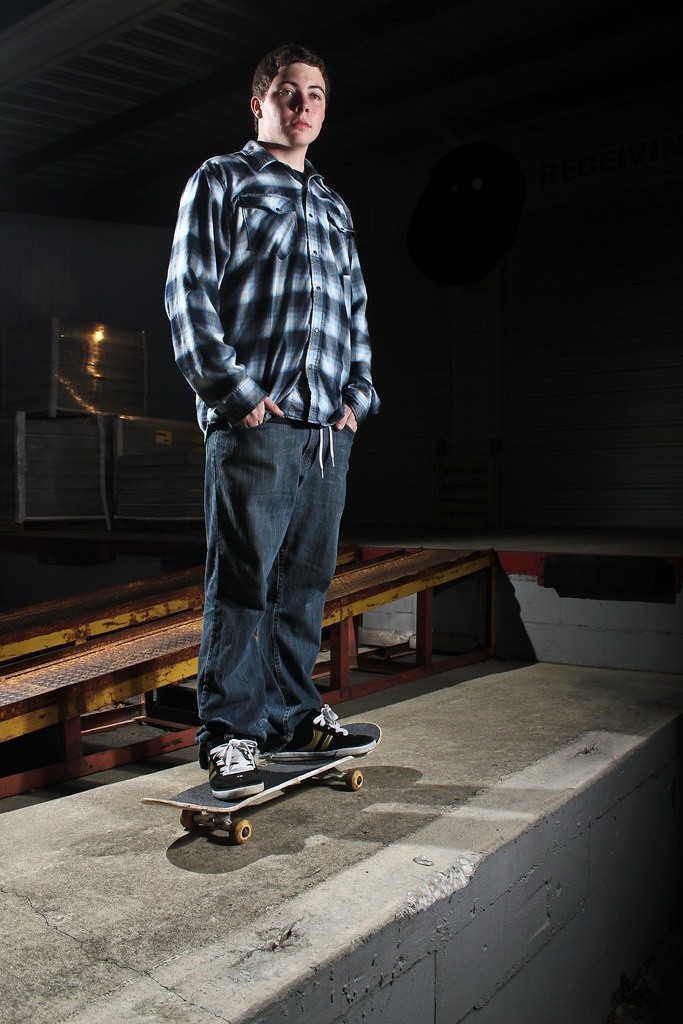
[141,717,385,840]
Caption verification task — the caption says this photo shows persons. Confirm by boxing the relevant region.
[162,41,386,800]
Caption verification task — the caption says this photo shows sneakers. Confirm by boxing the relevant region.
[207,733,265,799]
[269,704,376,762]
